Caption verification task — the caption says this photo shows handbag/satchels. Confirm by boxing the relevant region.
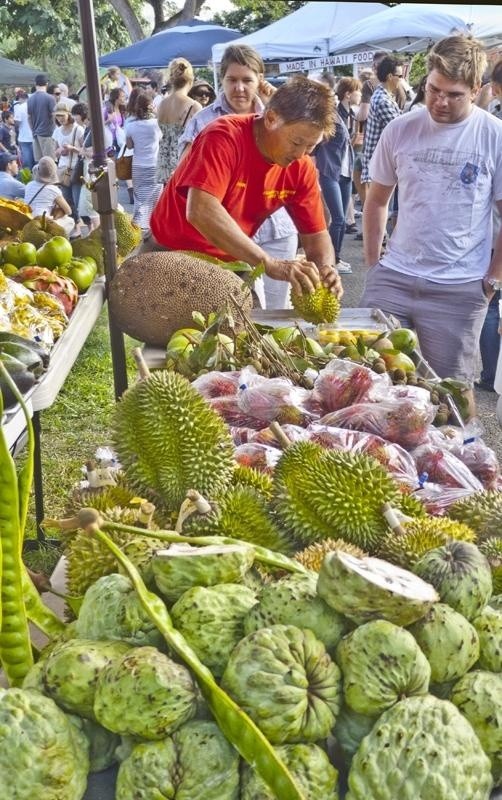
[61,156,85,187]
[115,144,134,180]
[8,101,21,114]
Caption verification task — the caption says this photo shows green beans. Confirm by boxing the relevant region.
[36,507,316,799]
[0,362,66,688]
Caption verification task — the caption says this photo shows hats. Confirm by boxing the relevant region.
[51,103,70,116]
[32,155,65,184]
[15,87,26,96]
[0,151,20,169]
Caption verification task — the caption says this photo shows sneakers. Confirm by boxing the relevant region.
[336,259,353,274]
[343,192,398,256]
[474,378,495,392]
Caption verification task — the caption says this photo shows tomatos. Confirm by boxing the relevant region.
[4,236,98,293]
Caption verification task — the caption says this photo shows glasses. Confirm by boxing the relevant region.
[421,78,477,104]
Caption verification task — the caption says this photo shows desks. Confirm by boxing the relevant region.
[2,255,111,552]
[139,305,443,391]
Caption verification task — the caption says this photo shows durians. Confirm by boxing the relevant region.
[57,346,501,598]
[288,280,342,323]
[115,210,140,258]
